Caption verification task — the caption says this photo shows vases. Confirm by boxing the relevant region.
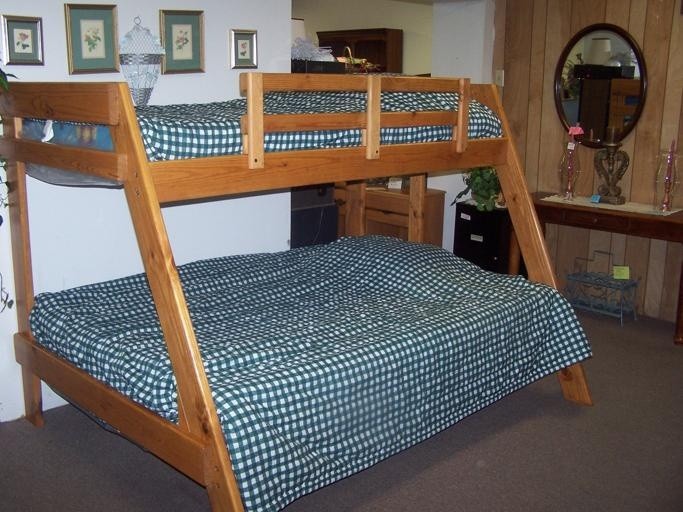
[557,140,581,201]
[654,148,680,213]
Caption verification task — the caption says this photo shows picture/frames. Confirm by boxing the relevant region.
[64,2,121,74]
[0,14,45,66]
[158,9,205,74]
[229,28,258,69]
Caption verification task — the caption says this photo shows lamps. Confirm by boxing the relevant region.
[291,17,305,48]
[587,37,612,67]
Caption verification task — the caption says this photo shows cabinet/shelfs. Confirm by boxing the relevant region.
[452,198,529,281]
[335,184,446,248]
[316,28,403,72]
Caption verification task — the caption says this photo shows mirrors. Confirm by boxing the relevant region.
[553,23,648,149]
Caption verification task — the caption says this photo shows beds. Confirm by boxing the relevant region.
[0,71,594,512]
[607,77,643,136]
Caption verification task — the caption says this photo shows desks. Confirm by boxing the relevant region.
[508,191,683,346]
